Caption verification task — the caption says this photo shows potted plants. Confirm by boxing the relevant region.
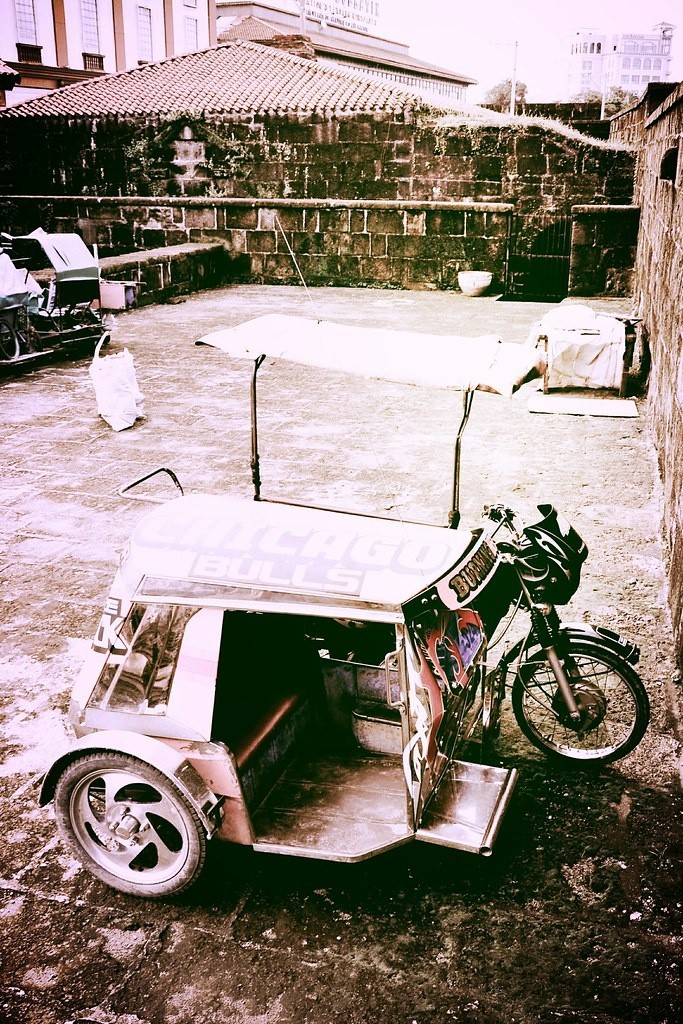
[458,237,492,298]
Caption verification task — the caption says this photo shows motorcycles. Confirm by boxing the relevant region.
[35,465,652,897]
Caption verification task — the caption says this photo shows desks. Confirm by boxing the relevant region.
[541,295,637,397]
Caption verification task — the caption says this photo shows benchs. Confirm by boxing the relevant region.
[38,307,84,319]
[233,689,313,811]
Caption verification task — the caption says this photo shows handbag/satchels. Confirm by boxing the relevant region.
[87,331,145,432]
